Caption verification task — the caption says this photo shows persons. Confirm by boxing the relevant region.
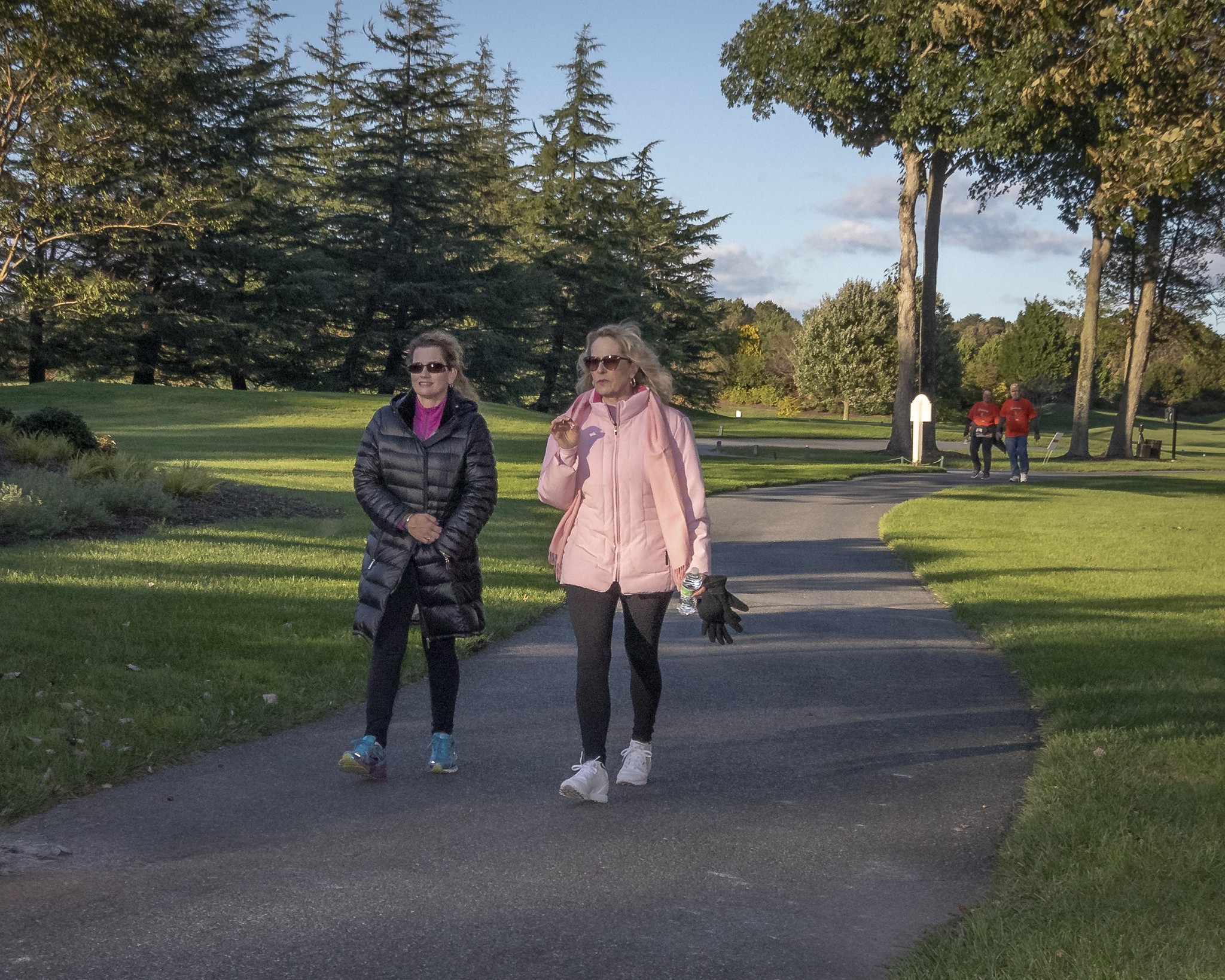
[338,329,497,780]
[963,388,1002,480]
[537,319,710,804]
[995,384,1040,482]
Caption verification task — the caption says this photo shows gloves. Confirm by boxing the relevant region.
[995,432,1003,442]
[1034,433,1041,440]
[696,576,751,645]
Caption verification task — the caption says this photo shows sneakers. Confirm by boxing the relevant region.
[1020,473,1028,482]
[428,732,458,773]
[559,758,610,803]
[981,473,990,480]
[616,740,654,786]
[338,734,389,780]
[970,468,982,478]
[1010,476,1020,482]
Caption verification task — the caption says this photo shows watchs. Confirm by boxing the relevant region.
[439,550,450,558]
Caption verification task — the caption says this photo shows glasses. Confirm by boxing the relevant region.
[408,361,448,373]
[584,355,630,372]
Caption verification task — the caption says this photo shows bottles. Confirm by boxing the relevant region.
[676,566,703,616]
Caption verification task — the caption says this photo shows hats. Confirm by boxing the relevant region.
[1009,383,1021,392]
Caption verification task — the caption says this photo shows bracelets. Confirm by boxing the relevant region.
[405,512,415,530]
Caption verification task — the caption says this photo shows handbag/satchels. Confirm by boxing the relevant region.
[973,425,988,437]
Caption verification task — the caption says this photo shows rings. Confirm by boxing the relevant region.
[427,536,431,540]
